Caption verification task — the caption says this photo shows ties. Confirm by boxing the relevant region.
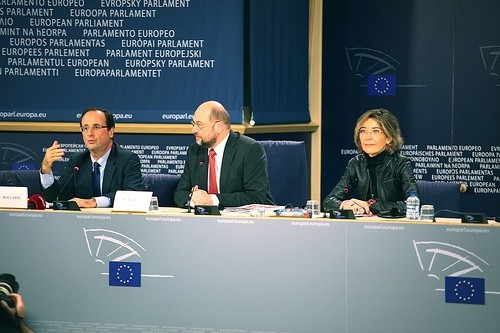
[93,162,101,197]
[208,149,218,194]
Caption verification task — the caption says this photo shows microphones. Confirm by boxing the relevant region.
[431,209,496,222]
[53,166,81,211]
[187,161,203,213]
[322,187,348,218]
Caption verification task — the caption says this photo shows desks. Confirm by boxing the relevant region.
[0,208,500,333]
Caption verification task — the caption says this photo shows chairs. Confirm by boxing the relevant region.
[256,139,310,205]
[142,174,180,207]
[416,180,459,218]
[0,170,40,198]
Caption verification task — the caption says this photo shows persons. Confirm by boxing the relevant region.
[322,108,418,216]
[174,101,276,209]
[39,108,142,208]
[0,293,34,333]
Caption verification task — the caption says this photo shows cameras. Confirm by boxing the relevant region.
[0,274,19,320]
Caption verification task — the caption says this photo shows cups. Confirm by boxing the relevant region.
[420,204,434,223]
[306,200,320,218]
[149,197,158,213]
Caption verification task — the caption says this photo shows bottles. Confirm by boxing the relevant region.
[405,190,420,221]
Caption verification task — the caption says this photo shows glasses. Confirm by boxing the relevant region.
[357,129,383,135]
[192,120,221,130]
[79,124,107,132]
